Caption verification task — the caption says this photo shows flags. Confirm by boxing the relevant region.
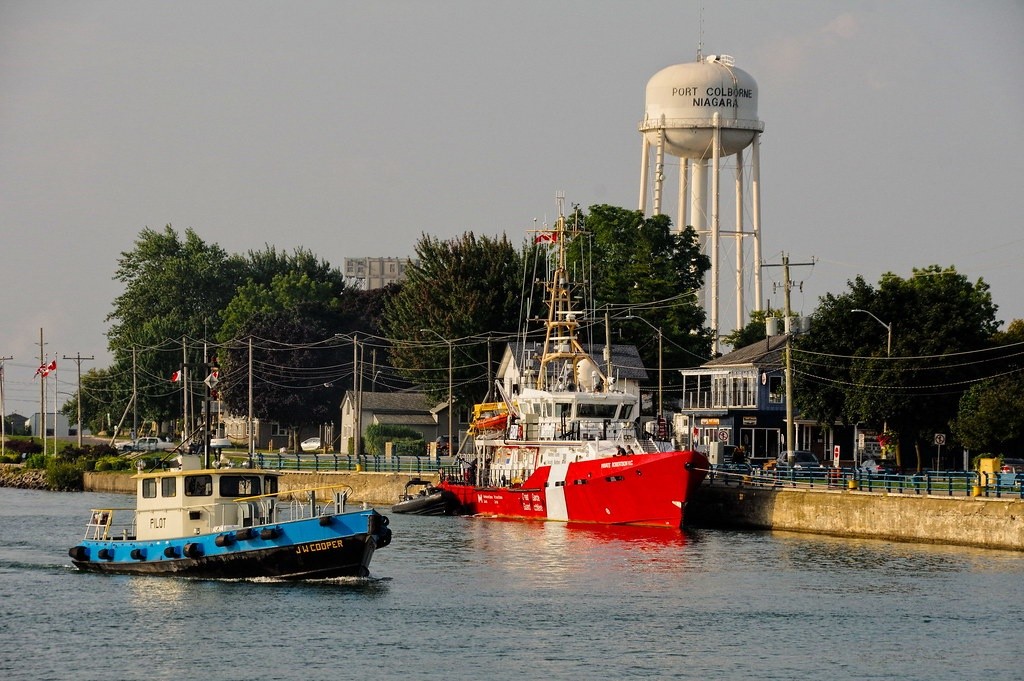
[171,370,182,383]
[34,359,57,378]
[533,232,558,246]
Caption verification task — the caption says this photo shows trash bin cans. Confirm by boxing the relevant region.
[979,457,1001,488]
[154,458,161,469]
[885,465,898,480]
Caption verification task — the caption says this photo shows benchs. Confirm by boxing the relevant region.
[995,474,1017,493]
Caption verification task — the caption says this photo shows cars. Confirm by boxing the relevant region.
[998,457,1024,491]
[437,433,459,458]
[279,437,333,454]
[853,458,904,485]
[114,436,175,453]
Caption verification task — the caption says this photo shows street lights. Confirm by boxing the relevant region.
[420,328,453,458]
[849,308,892,435]
[626,315,664,420]
[334,334,364,456]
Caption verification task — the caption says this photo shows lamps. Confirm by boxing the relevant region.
[134,459,147,474]
[212,459,220,469]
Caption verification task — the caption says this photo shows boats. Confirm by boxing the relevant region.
[66,362,462,586]
[427,189,713,532]
[391,474,468,519]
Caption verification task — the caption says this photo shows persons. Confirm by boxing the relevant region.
[616,445,634,456]
[596,382,602,392]
[424,485,428,496]
[197,436,204,455]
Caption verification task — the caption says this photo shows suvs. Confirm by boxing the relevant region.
[698,445,751,474]
[777,450,825,480]
